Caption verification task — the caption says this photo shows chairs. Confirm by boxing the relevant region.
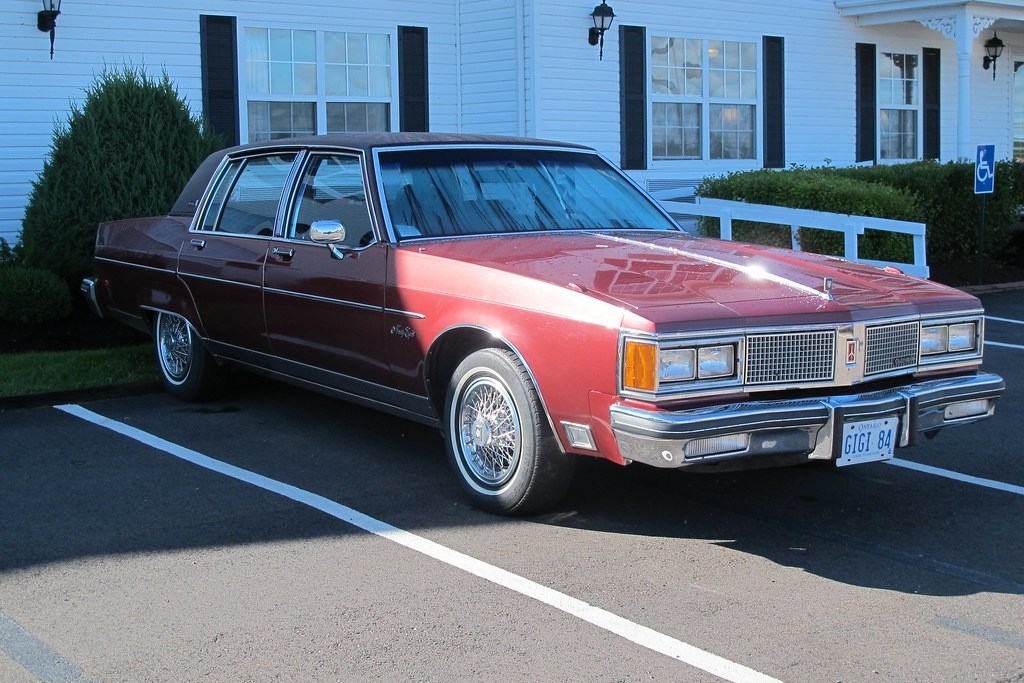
[394,183,425,225]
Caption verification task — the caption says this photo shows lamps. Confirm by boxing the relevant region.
[588,0,618,61]
[982,29,1006,81]
[37,0,62,60]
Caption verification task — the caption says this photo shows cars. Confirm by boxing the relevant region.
[75,132,1006,518]
